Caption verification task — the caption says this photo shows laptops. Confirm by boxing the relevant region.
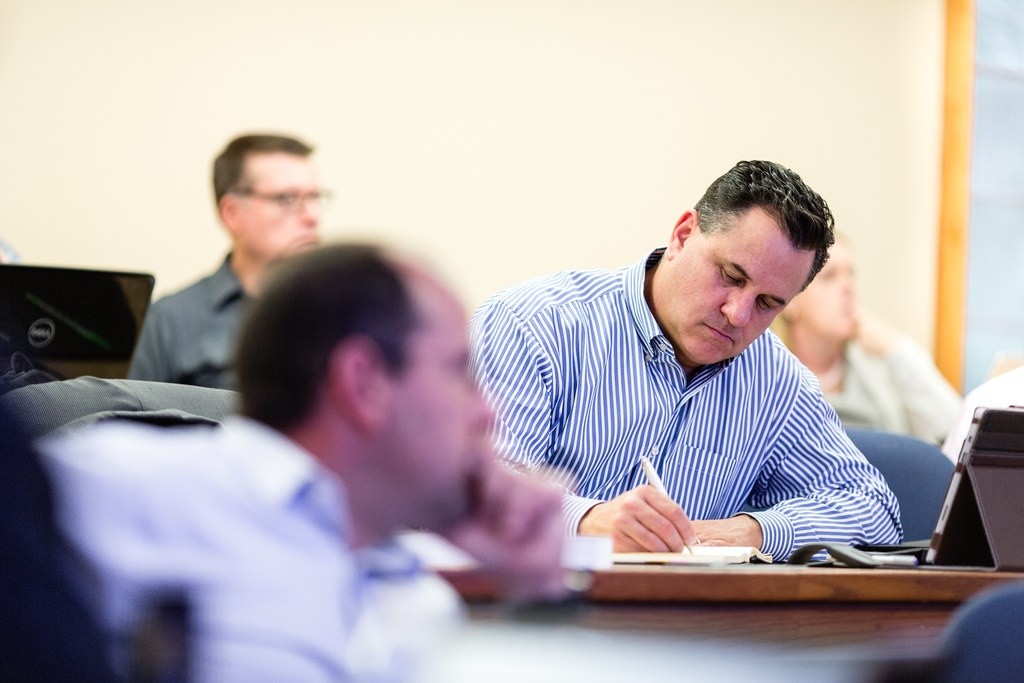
[0,264,156,381]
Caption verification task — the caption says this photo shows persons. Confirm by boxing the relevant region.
[121,132,333,394]
[781,226,964,446]
[27,238,589,683]
[456,159,905,555]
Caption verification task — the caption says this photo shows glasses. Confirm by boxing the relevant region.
[231,187,327,208]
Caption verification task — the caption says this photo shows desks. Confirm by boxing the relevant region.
[397,527,1024,683]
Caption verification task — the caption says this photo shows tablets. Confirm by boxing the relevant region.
[925,407,1024,564]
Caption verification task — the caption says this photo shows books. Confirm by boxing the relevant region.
[611,543,775,567]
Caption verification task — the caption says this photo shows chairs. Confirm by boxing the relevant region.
[843,428,961,544]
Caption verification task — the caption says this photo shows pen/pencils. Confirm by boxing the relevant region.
[639,455,696,555]
